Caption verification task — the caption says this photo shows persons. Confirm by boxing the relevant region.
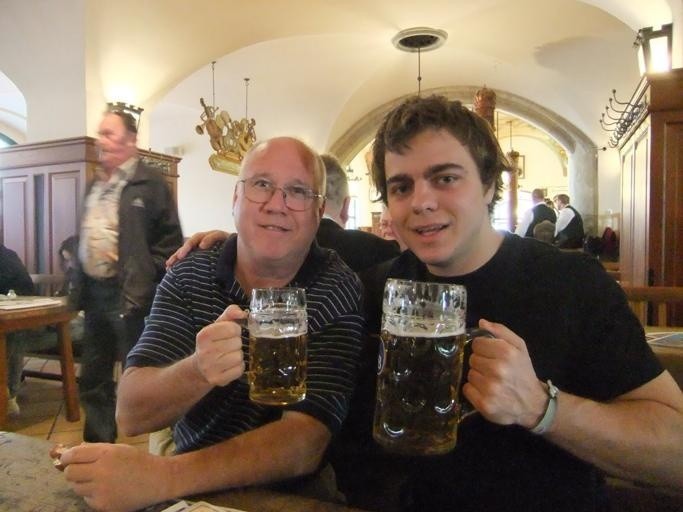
[513,187,612,264]
[71,112,186,444]
[164,94,681,511]
[376,205,408,254]
[58,133,365,511]
[5,235,86,421]
[316,151,399,276]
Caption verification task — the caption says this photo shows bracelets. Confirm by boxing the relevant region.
[524,376,561,439]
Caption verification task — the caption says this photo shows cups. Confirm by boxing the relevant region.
[370,275,498,459]
[221,284,310,409]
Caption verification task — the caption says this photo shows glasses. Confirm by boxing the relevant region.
[239,176,325,211]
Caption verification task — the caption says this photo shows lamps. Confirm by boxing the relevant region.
[633,22,677,79]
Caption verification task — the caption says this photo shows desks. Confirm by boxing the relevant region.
[0,428,364,510]
[641,324,682,386]
[0,295,79,429]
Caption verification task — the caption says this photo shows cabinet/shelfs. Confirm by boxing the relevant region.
[0,134,181,296]
[613,65,683,326]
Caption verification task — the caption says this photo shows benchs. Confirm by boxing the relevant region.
[0,274,126,388]
